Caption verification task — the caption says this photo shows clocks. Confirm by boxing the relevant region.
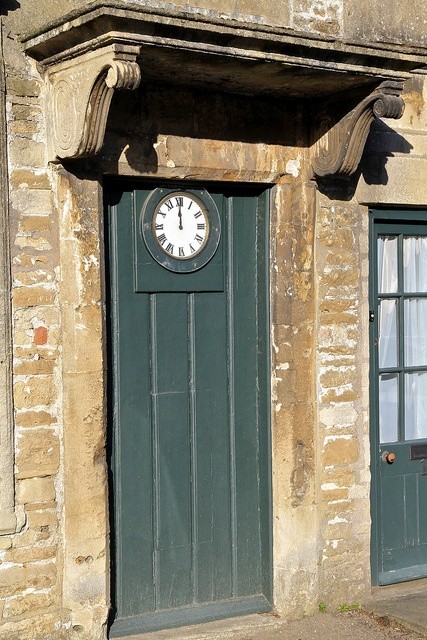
[151,191,211,262]
